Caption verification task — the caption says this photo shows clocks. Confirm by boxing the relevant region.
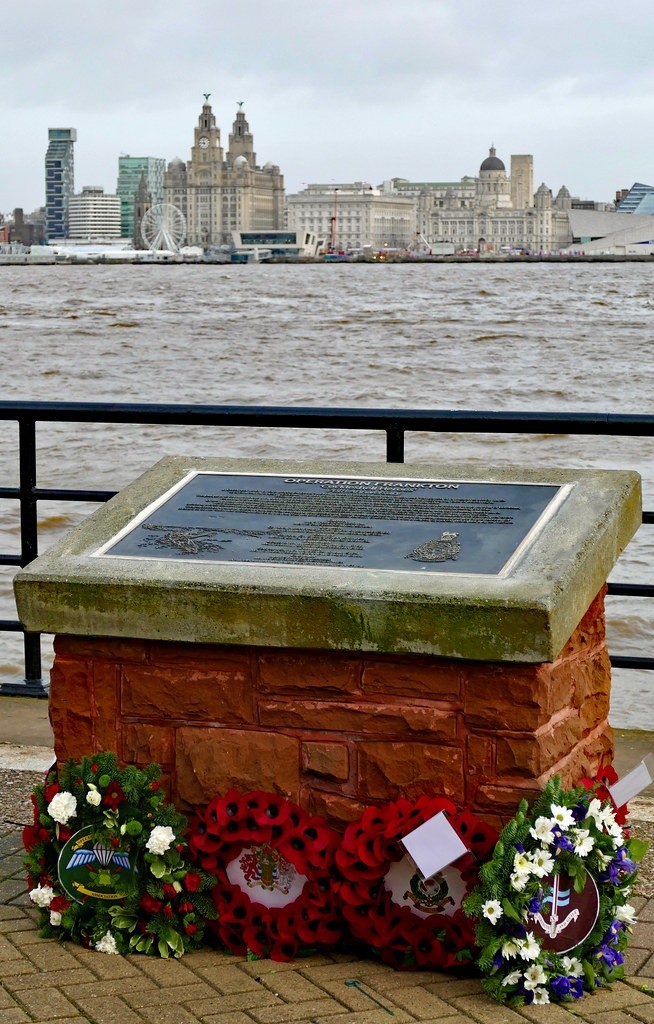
[198,136,210,149]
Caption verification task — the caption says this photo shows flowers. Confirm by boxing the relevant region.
[462,772,637,1007]
[20,749,212,957]
[184,792,345,964]
[331,793,500,972]
[578,757,631,845]
